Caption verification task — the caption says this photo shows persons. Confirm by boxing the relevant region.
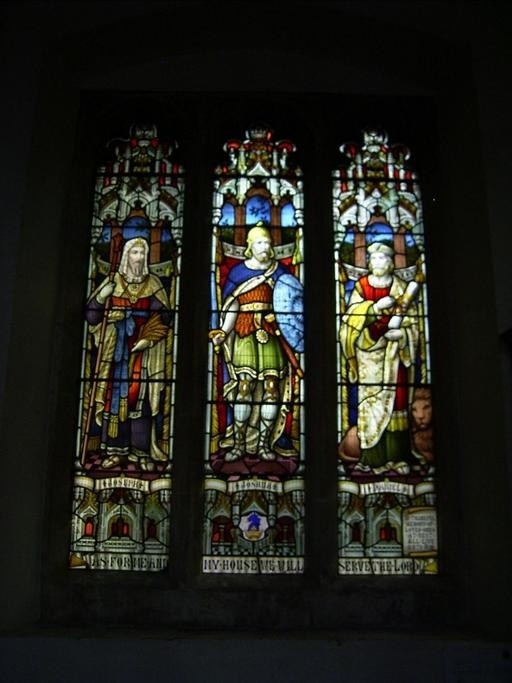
[340,241,420,477]
[84,236,170,468]
[209,220,304,462]
